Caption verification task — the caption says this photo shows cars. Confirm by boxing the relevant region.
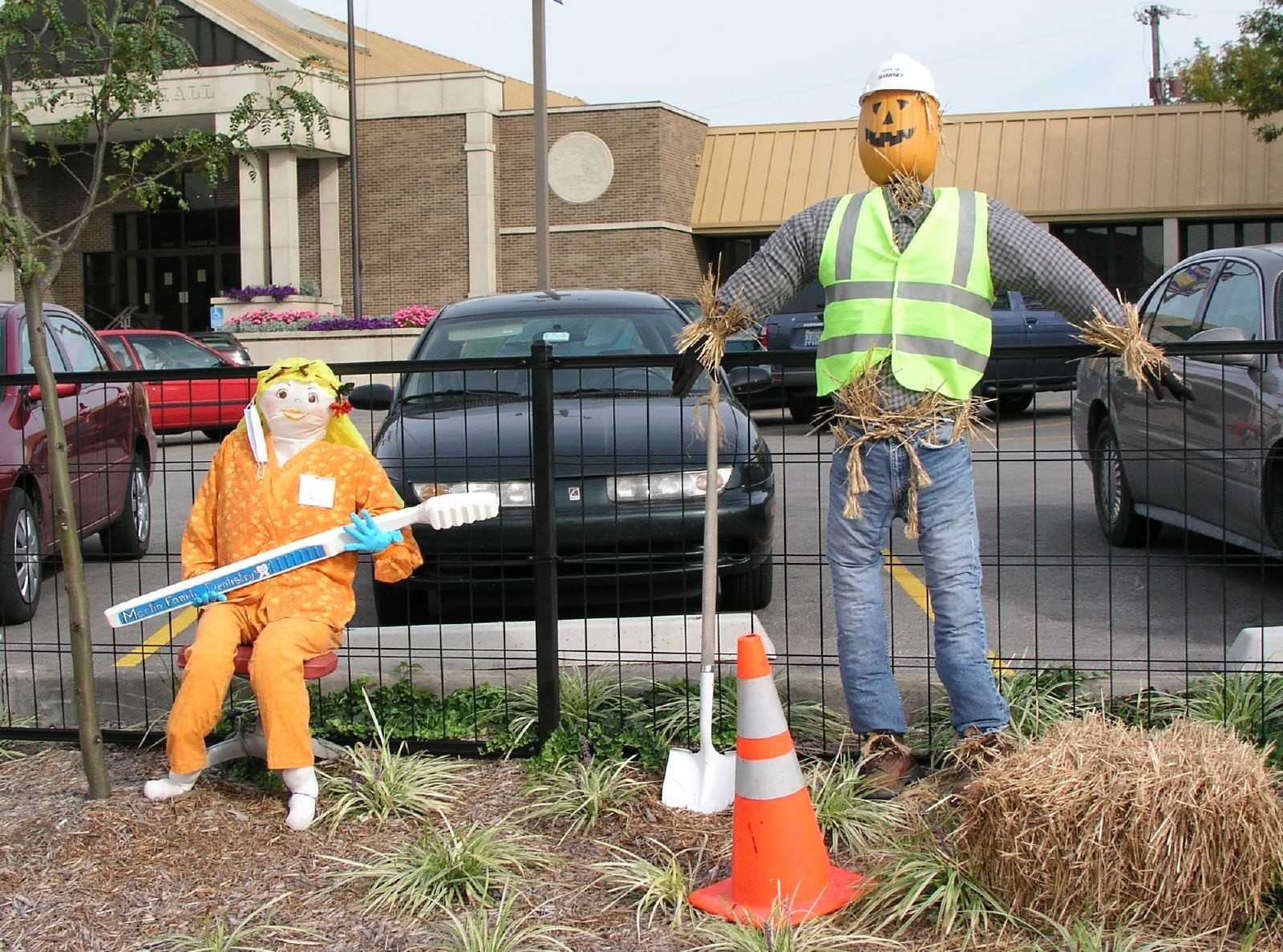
[85,331,270,442]
[764,276,1107,431]
[1,297,158,627]
[656,296,770,414]
[346,288,774,616]
[1071,239,1283,563]
[190,332,252,368]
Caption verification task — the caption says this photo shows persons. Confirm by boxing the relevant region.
[143,358,423,830]
[668,56,1196,800]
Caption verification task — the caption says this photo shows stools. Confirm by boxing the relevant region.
[175,643,350,772]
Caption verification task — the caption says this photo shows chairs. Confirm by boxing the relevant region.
[589,348,660,389]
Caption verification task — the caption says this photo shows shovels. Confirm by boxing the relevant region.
[660,369,742,819]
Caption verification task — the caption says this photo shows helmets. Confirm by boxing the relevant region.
[859,51,942,107]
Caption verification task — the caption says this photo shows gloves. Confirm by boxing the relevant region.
[192,590,227,606]
[343,509,403,553]
[670,333,711,398]
[1141,361,1196,402]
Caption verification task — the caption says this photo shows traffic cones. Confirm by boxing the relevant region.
[688,634,880,936]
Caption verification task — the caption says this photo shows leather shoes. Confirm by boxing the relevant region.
[855,746,925,800]
